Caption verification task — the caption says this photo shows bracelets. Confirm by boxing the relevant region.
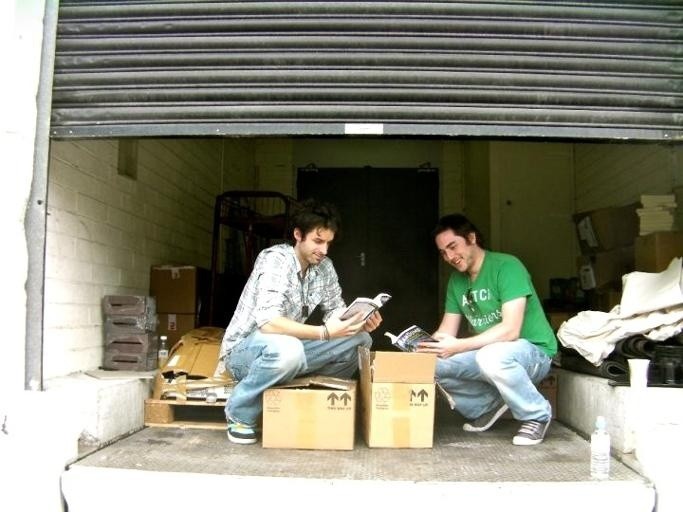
[319,322,330,344]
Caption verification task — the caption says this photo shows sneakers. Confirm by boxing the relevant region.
[512,418,551,445]
[463,402,509,432]
[224,408,258,444]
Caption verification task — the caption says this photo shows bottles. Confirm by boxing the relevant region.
[589,416,610,484]
[147,335,170,370]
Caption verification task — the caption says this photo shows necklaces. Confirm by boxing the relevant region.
[299,268,310,318]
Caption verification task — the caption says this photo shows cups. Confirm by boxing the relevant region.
[627,357,649,389]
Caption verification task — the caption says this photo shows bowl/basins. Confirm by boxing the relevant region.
[656,345,682,384]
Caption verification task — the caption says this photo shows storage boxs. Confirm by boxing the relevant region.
[261,374,358,451]
[149,263,210,349]
[545,200,683,333]
[357,345,438,450]
[500,371,558,422]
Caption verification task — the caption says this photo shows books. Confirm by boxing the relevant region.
[340,292,391,324]
[384,325,438,352]
[636,192,677,238]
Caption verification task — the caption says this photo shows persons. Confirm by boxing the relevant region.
[415,212,559,448]
[216,198,383,444]
